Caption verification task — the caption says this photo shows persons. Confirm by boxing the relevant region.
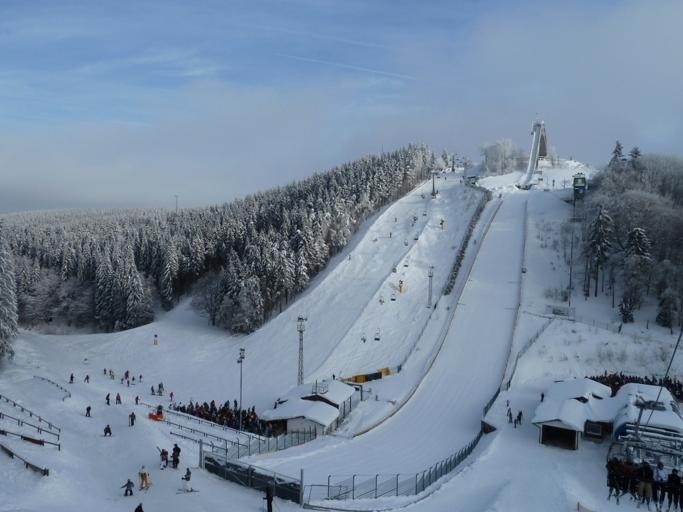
[175,399,277,437]
[68,366,174,436]
[507,392,545,428]
[122,443,192,512]
[606,456,683,512]
